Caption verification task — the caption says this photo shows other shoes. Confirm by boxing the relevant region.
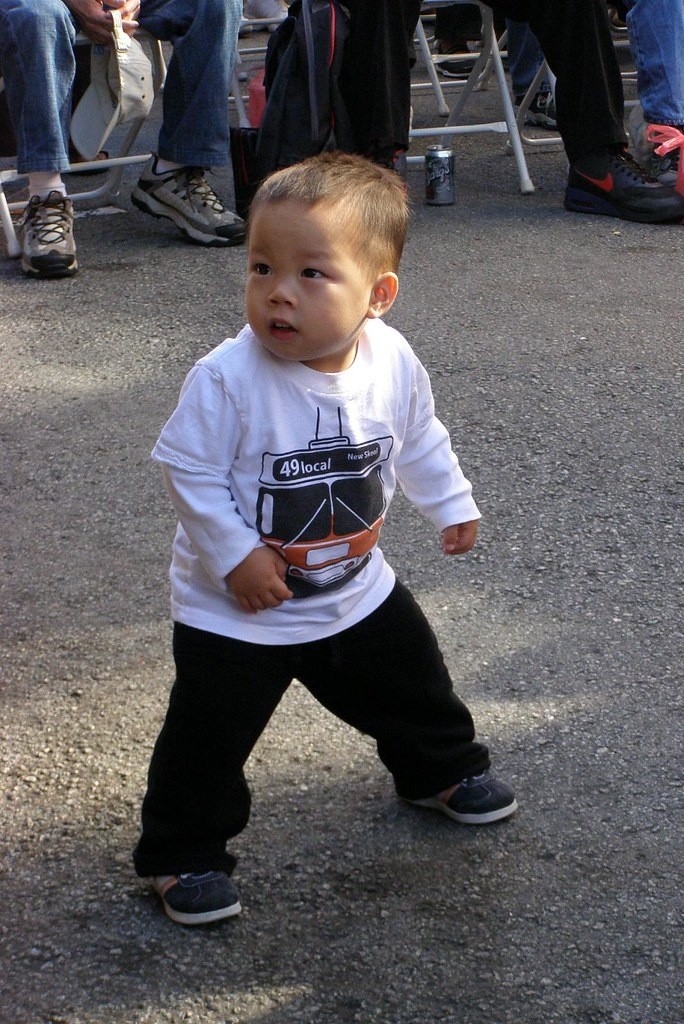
[407,769,518,823]
[649,129,683,187]
[149,872,242,923]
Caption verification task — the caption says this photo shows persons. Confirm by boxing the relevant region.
[131,151,519,926]
[0,0,684,280]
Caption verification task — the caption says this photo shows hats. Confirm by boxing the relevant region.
[71,10,153,160]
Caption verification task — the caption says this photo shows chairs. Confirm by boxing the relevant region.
[0,0,639,258]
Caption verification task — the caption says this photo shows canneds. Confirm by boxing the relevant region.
[425,144,454,206]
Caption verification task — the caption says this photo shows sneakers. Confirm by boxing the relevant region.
[512,91,559,129]
[16,190,79,281]
[130,151,248,246]
[562,147,684,225]
[435,41,476,78]
